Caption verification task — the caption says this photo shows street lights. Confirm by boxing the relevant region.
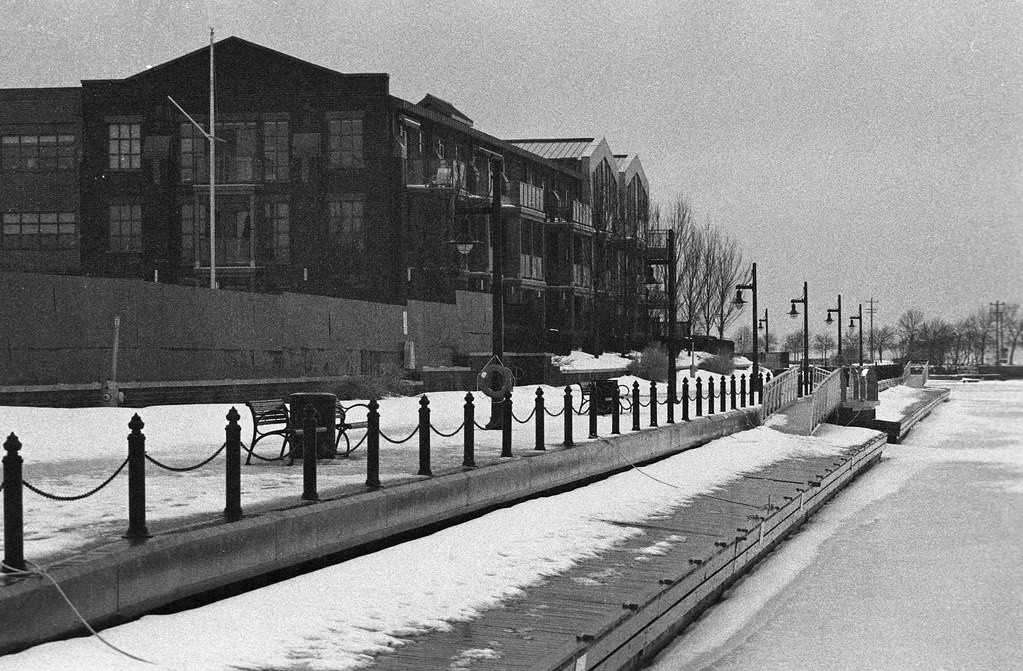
[758,308,768,351]
[449,160,504,429]
[825,295,841,354]
[640,229,678,403]
[732,263,759,392]
[849,304,863,365]
[787,283,808,383]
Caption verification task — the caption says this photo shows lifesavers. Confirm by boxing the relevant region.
[479,364,512,399]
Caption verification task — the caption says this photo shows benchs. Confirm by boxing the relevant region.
[334,394,372,457]
[577,380,633,416]
[245,399,328,466]
